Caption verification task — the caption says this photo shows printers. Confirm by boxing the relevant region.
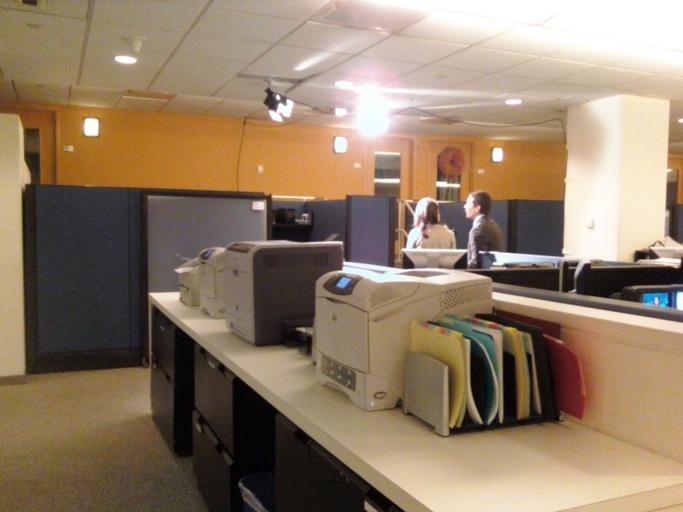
[315,268,492,411]
[174,256,200,306]
[200,246,227,320]
[224,239,344,347]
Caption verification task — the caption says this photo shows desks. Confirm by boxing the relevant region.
[146,292,683,512]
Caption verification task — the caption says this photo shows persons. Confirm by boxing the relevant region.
[462,191,502,267]
[405,198,458,250]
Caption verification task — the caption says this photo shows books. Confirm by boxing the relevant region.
[411,308,545,426]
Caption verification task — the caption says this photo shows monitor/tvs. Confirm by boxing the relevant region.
[624,285,683,311]
[401,248,468,269]
